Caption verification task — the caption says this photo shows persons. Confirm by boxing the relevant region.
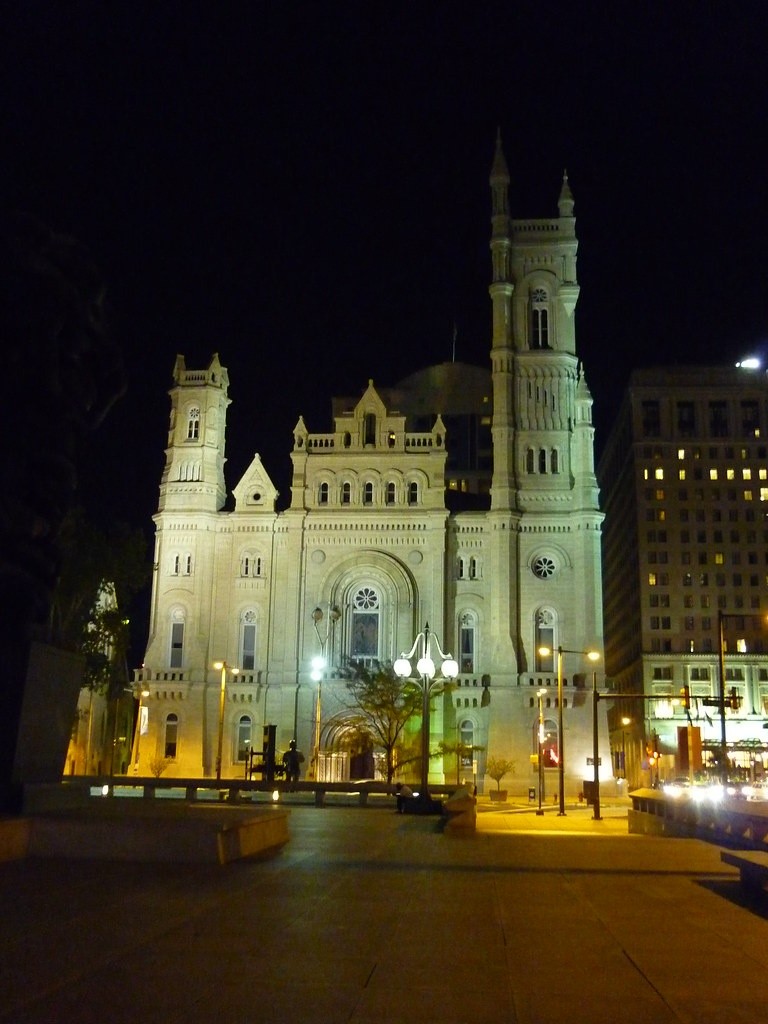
[283,739,304,781]
[394,782,413,814]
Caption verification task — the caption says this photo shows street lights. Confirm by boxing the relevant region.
[312,604,341,657]
[213,662,239,790]
[622,716,654,787]
[536,688,547,815]
[127,690,150,776]
[309,656,326,782]
[393,620,459,798]
[539,645,602,816]
